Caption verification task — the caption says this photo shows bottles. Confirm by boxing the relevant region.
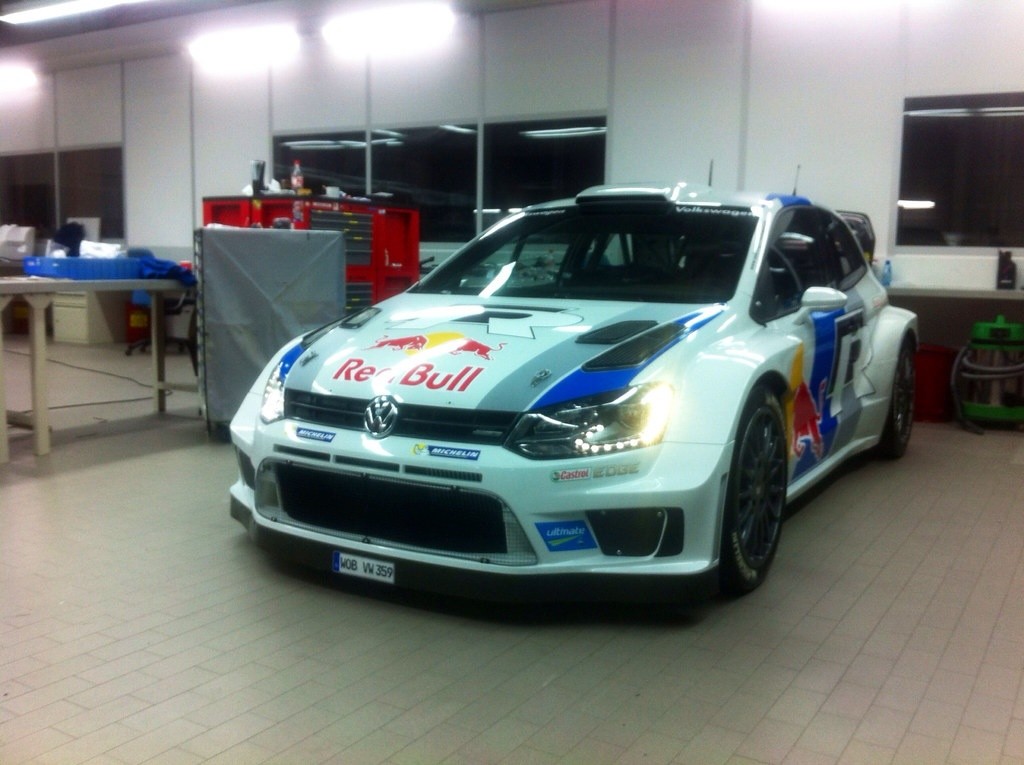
[871,258,892,286]
[996,251,1017,289]
[291,159,304,191]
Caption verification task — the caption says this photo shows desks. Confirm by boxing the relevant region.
[0,277,199,464]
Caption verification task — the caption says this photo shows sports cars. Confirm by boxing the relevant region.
[230,181,920,602]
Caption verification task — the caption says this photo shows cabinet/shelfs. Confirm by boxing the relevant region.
[52,291,129,344]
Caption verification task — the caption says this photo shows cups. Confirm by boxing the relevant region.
[326,186,340,197]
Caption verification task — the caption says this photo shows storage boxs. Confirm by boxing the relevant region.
[23,256,141,279]
